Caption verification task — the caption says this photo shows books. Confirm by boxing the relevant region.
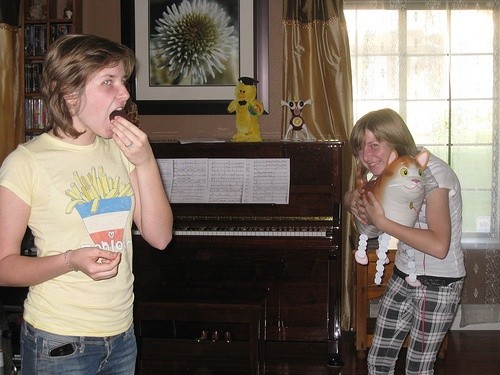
[22,22,68,143]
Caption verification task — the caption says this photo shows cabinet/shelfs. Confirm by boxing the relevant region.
[19,0,83,143]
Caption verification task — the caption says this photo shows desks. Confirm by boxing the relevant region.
[349,235,448,369]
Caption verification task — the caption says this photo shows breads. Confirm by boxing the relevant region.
[109,109,141,127]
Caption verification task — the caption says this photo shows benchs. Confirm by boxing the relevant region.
[2,286,266,375]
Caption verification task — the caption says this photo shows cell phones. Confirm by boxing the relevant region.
[48,341,75,356]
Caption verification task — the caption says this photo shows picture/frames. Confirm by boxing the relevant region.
[120,0,270,115]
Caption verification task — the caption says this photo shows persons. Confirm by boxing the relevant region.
[0,35,174,375]
[345,109,466,375]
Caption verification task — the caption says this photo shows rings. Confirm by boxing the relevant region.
[126,142,133,148]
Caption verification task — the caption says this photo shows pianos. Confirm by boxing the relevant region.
[0,137,345,375]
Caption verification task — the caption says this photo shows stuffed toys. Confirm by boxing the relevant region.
[227,77,263,142]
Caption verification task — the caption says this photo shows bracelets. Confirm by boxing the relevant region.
[65,248,78,274]
